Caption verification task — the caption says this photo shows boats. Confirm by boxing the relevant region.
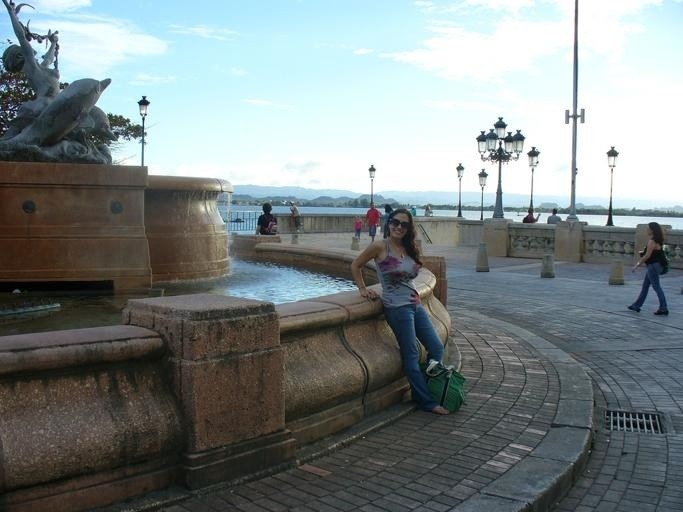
[223,216,246,223]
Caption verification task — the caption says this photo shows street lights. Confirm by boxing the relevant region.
[137,96,149,166]
[476,167,489,220]
[527,146,540,208]
[367,165,376,202]
[476,115,524,219]
[455,163,464,216]
[605,146,618,226]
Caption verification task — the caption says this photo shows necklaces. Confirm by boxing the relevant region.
[388,237,405,259]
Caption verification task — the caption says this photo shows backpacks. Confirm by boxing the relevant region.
[260,214,278,235]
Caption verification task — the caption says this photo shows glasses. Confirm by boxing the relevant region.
[388,218,412,229]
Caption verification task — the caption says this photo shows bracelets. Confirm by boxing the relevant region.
[636,261,640,267]
[357,286,367,292]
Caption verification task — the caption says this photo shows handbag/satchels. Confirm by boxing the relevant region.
[660,250,669,274]
[420,359,467,413]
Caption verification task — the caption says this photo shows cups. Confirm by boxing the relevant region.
[638,251,642,257]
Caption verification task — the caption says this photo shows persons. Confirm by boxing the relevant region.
[252,202,279,236]
[626,221,671,316]
[522,207,541,223]
[546,208,561,224]
[0,1,62,142]
[288,200,303,233]
[349,207,453,417]
[349,202,434,243]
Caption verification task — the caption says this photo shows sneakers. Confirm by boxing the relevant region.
[628,306,640,312]
[654,310,668,315]
